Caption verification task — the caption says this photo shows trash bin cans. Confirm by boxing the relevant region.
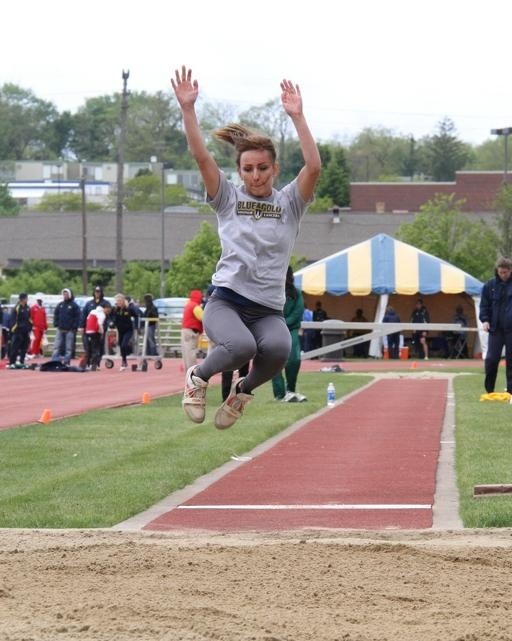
[320,319,347,362]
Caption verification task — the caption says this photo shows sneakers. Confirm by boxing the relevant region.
[274,390,310,402]
[215,377,255,430]
[181,366,209,422]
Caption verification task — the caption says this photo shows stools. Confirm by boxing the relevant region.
[437,324,470,359]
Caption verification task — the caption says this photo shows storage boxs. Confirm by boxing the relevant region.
[382,335,405,347]
[381,346,410,360]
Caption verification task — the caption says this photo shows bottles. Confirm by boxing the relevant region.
[327,383,335,407]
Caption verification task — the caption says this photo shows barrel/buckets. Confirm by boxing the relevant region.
[398,346,410,360]
[382,345,390,360]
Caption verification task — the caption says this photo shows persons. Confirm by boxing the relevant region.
[452,304,470,358]
[170,63,321,429]
[479,257,512,395]
[410,299,429,360]
[347,309,372,357]
[0,287,158,371]
[382,305,400,358]
[180,266,331,402]
